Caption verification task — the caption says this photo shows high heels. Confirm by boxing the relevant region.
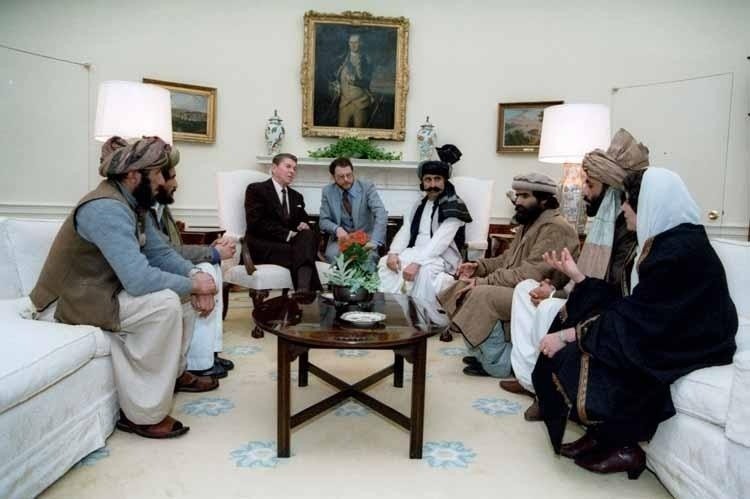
[572,442,648,481]
[552,434,608,457]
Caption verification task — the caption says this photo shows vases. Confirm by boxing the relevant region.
[330,281,378,303]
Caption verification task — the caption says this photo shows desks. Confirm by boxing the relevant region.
[485,219,587,259]
[250,286,454,460]
[179,224,227,248]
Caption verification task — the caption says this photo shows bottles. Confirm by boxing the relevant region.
[265,109,285,157]
[416,116,438,163]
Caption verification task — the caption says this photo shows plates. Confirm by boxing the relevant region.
[339,311,386,327]
[320,291,334,303]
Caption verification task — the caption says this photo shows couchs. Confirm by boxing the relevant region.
[0,214,121,497]
[620,235,750,499]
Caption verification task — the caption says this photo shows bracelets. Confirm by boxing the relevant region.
[559,331,566,344]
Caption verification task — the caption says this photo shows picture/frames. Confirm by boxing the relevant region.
[298,7,412,145]
[141,76,219,146]
[495,99,566,155]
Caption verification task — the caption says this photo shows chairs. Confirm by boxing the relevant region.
[214,168,499,340]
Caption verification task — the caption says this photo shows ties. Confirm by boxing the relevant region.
[281,188,289,216]
[341,190,352,215]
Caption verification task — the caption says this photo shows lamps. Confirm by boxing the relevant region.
[536,101,614,234]
[91,79,174,148]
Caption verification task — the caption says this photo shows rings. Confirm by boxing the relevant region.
[544,347,547,353]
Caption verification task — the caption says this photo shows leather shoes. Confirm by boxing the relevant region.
[461,365,488,379]
[461,354,478,365]
[439,329,453,343]
[496,375,532,397]
[212,352,235,370]
[522,398,543,424]
[187,367,229,380]
[476,374,485,376]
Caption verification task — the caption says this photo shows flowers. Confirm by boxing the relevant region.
[320,227,384,296]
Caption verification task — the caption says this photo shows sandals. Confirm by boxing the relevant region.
[174,372,220,394]
[113,407,191,441]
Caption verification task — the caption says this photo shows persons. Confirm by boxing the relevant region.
[438,172,580,377]
[318,158,389,276]
[498,128,649,418]
[538,166,737,481]
[326,33,372,128]
[148,138,236,380]
[376,159,472,343]
[238,154,323,296]
[25,137,217,439]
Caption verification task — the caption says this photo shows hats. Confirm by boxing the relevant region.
[415,159,455,180]
[166,143,182,169]
[510,170,558,193]
[581,147,625,190]
[97,131,172,178]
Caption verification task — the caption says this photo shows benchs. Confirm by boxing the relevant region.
[242,214,405,266]
